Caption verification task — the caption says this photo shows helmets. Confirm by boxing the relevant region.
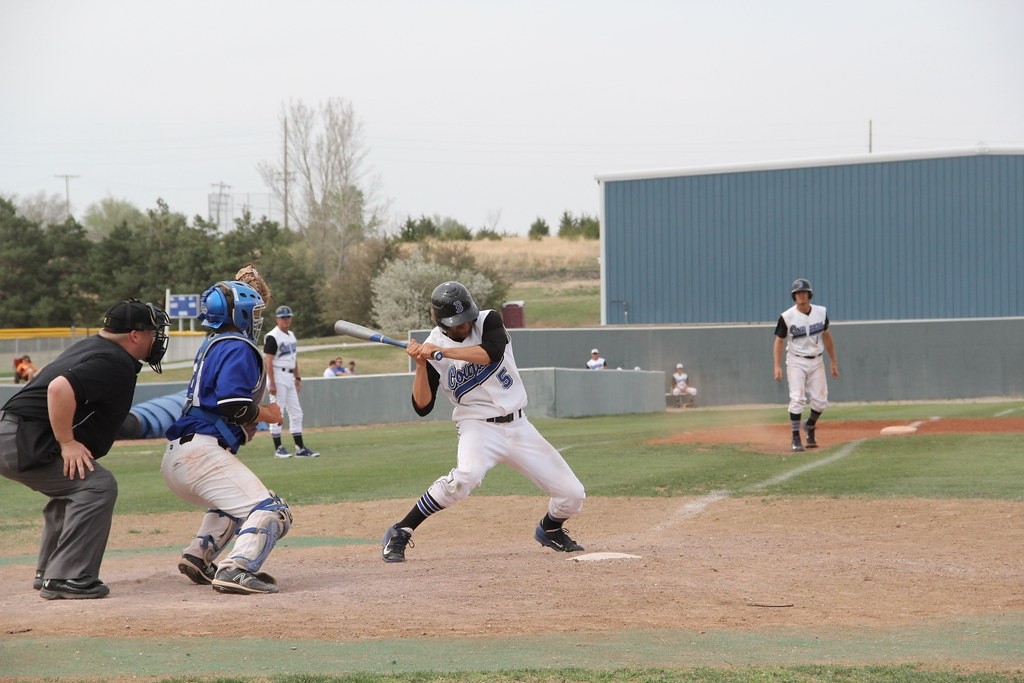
[197,279,267,345]
[431,281,479,327]
[790,278,812,301]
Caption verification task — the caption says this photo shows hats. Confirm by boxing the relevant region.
[102,298,170,333]
[677,364,684,368]
[276,306,294,318]
[590,349,598,354]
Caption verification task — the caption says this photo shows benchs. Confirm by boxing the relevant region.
[665,393,688,408]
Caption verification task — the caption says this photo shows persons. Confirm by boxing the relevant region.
[323,358,358,378]
[586,348,641,370]
[263,305,320,457]
[773,278,839,452]
[380,281,586,560]
[17,355,38,382]
[670,362,697,408]
[162,266,293,598]
[0,299,154,600]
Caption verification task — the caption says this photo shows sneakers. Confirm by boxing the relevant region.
[33,570,47,589]
[178,554,216,584]
[381,523,415,563]
[296,448,321,457]
[791,437,804,450]
[535,519,585,552]
[212,568,280,595]
[274,445,294,458]
[39,575,109,600]
[806,429,817,448]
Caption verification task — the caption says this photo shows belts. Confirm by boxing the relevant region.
[796,353,823,359]
[169,435,228,450]
[487,408,521,423]
[282,368,294,373]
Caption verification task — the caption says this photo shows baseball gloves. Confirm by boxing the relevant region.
[233,264,272,309]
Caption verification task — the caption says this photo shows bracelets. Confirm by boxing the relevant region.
[296,377,301,381]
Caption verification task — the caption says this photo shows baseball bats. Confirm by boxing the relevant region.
[333,319,444,361]
